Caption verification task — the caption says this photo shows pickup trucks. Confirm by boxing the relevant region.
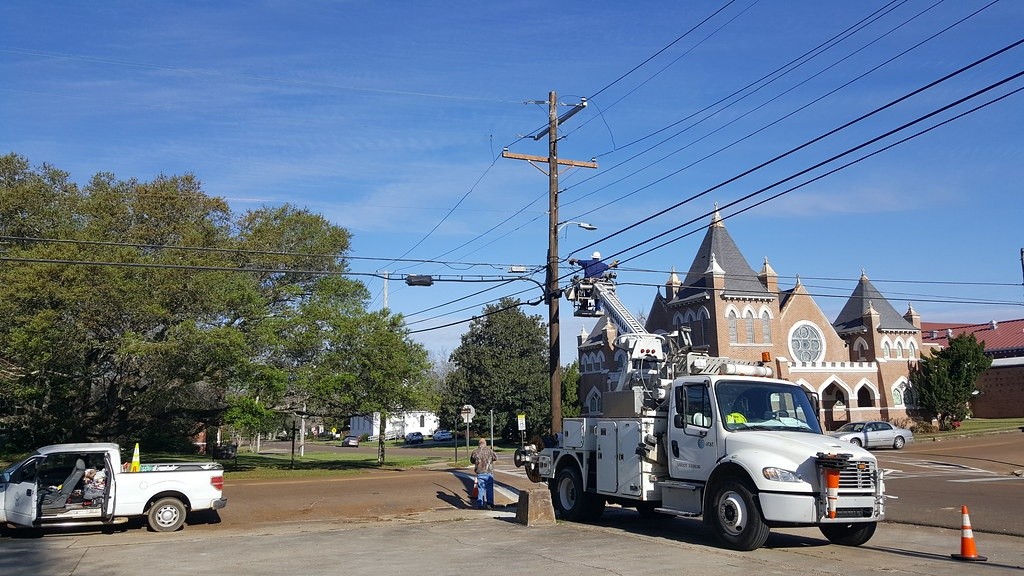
[0,443,227,532]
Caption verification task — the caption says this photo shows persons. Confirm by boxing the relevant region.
[570,251,619,312]
[470,438,497,510]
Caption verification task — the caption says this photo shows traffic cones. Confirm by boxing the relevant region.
[129,443,140,472]
[467,477,478,499]
[951,505,988,561]
[821,454,848,519]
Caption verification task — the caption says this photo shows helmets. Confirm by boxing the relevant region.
[591,252,601,258]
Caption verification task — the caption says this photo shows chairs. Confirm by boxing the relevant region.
[687,415,711,426]
[38,459,85,509]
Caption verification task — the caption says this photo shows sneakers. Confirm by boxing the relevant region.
[487,504,494,509]
[478,505,484,508]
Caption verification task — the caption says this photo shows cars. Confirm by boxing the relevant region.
[343,435,358,440]
[405,432,423,445]
[433,430,453,441]
[318,432,328,437]
[342,437,359,447]
[824,421,914,450]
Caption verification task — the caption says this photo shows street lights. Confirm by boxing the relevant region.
[552,221,598,441]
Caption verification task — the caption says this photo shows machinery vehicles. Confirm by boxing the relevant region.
[513,274,885,553]
